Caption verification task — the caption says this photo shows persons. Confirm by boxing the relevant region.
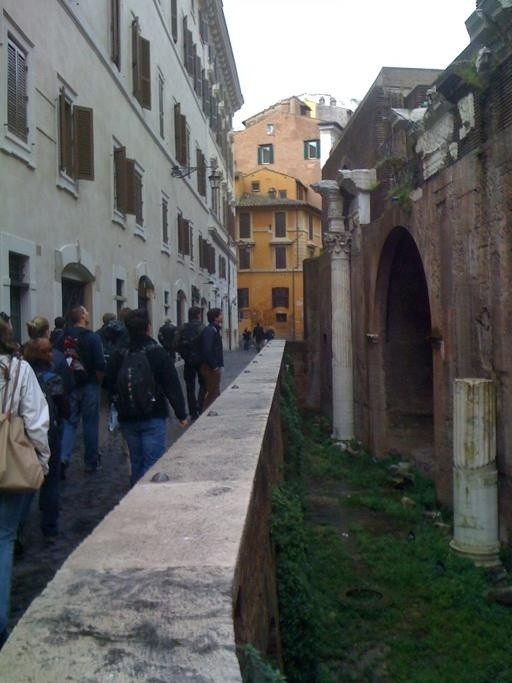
[242,322,276,353]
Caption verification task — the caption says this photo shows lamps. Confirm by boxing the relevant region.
[170,164,222,191]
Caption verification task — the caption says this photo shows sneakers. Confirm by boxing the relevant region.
[59,460,70,481]
[43,529,64,543]
[85,453,102,472]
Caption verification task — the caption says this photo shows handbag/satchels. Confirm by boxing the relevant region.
[0,357,45,495]
[35,370,72,400]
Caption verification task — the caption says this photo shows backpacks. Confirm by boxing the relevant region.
[178,321,204,367]
[99,329,128,389]
[60,330,93,387]
[113,343,163,422]
